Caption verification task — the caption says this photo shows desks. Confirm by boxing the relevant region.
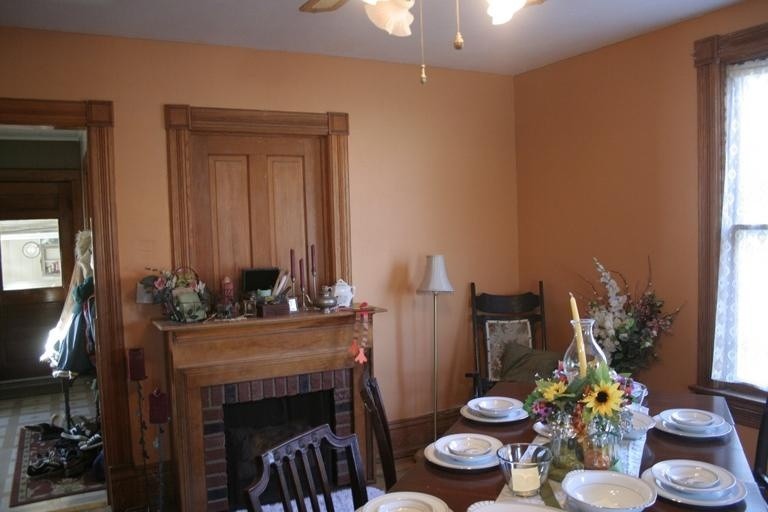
[49,363,100,430]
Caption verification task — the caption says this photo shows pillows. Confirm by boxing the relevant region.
[497,341,562,384]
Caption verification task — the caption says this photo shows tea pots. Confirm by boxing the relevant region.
[304,286,338,314]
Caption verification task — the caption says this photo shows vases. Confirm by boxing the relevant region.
[548,412,618,473]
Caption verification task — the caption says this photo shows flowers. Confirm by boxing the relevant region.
[521,358,638,422]
[571,255,675,376]
[135,259,207,308]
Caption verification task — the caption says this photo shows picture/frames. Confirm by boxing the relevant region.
[286,296,300,315]
[243,299,258,317]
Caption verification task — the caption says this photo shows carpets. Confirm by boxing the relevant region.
[7,423,107,508]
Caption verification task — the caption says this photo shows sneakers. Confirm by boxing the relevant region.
[78,433,103,451]
[25,450,68,479]
[60,421,92,441]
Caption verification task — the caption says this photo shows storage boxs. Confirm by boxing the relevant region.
[255,303,289,320]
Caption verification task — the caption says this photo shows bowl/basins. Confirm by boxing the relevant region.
[608,409,656,441]
[562,468,658,511]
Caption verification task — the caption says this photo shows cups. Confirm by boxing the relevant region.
[632,382,647,411]
[497,442,554,497]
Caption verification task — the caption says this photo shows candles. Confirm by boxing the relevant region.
[299,257,305,288]
[310,244,317,272]
[289,248,295,277]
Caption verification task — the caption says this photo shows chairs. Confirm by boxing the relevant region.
[469,278,551,405]
[360,373,395,486]
[752,391,768,503]
[246,422,372,510]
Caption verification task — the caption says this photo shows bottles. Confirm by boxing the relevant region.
[562,319,608,383]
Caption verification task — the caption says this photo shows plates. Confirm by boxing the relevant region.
[459,396,529,422]
[423,433,504,471]
[641,459,747,507]
[353,491,453,512]
[466,500,563,511]
[651,407,733,439]
[532,420,574,438]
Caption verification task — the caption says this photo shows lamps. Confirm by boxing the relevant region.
[347,0,533,89]
[415,250,456,444]
